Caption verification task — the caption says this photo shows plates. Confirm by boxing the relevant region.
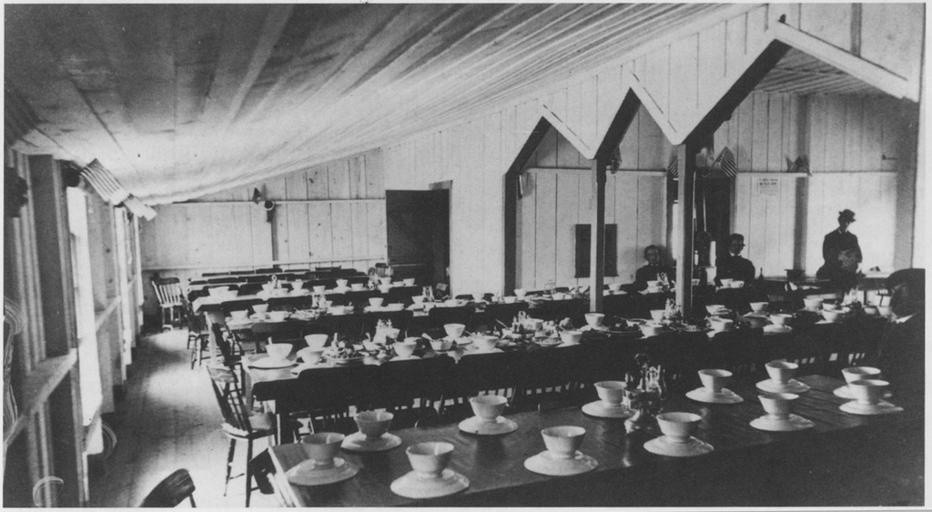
[686,387,744,404]
[747,415,815,431]
[458,415,519,436]
[228,319,253,326]
[524,448,599,475]
[339,431,403,451]
[644,434,715,456]
[832,386,898,415]
[583,400,634,418]
[290,366,302,374]
[286,456,359,486]
[254,357,292,369]
[754,378,810,394]
[388,469,471,498]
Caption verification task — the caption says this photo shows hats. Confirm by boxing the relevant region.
[840,210,855,221]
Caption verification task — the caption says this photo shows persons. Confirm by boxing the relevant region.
[718,233,755,273]
[636,245,675,282]
[865,268,925,367]
[823,209,863,273]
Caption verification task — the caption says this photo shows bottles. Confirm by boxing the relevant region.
[624,355,669,416]
[423,285,435,312]
[758,267,764,288]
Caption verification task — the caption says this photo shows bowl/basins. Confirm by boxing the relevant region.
[230,305,268,322]
[541,425,586,456]
[594,381,626,403]
[405,440,455,477]
[697,368,731,391]
[353,409,394,438]
[656,413,702,442]
[514,278,745,299]
[265,297,890,364]
[301,431,345,465]
[764,361,798,382]
[757,393,801,419]
[840,366,889,405]
[209,275,416,299]
[469,395,507,420]
